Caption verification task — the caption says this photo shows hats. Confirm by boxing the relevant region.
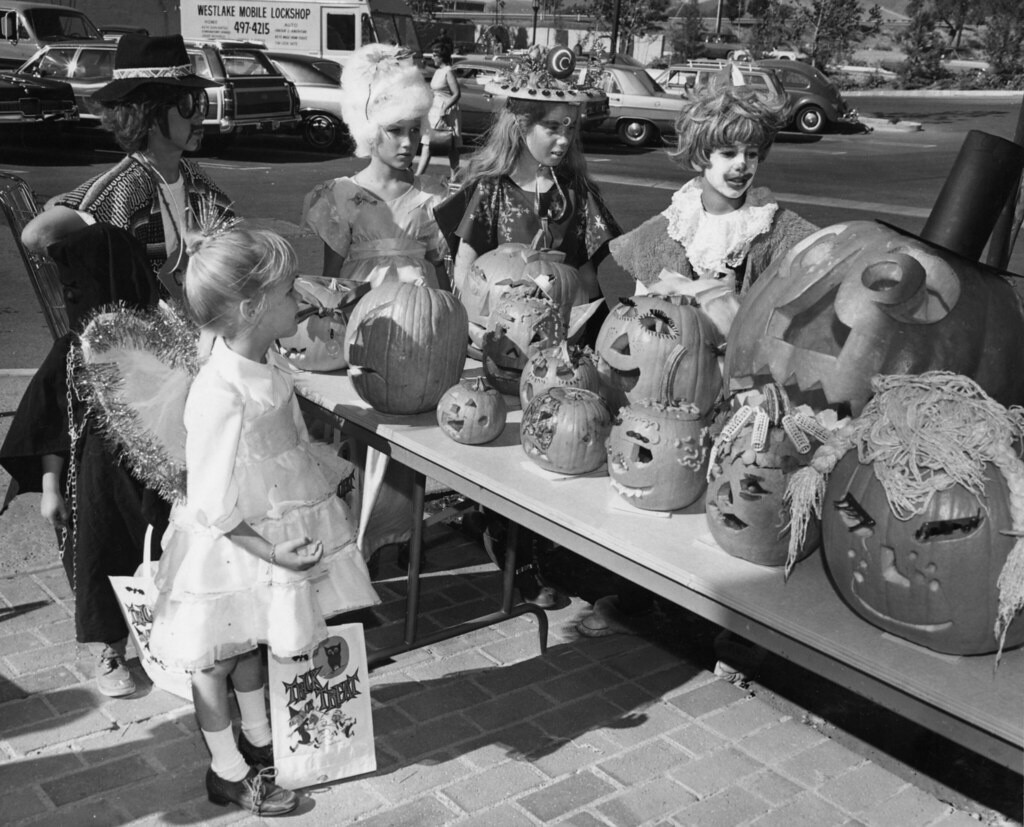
[484,43,609,103]
[91,32,223,102]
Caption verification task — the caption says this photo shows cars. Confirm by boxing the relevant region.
[436,59,609,148]
[567,62,692,151]
[755,59,870,141]
[654,64,786,103]
[1,67,80,154]
[262,48,347,149]
[18,39,301,157]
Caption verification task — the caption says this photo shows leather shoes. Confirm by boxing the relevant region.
[237,729,274,774]
[205,765,300,817]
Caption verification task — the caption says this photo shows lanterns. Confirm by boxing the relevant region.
[706,406,823,567]
[594,285,723,418]
[461,229,585,362]
[725,129,1024,420]
[272,274,363,374]
[518,385,612,475]
[481,273,565,396]
[605,344,709,512]
[435,376,507,446]
[342,276,470,414]
[520,340,599,410]
[782,369,1024,668]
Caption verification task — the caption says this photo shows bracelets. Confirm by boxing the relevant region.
[270,543,278,567]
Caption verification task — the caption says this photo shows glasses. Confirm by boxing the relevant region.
[167,88,210,119]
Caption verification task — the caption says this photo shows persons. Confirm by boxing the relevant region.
[44,35,237,289]
[150,198,380,815]
[433,48,623,606]
[580,85,825,678]
[304,45,454,571]
[0,221,170,700]
[414,45,461,181]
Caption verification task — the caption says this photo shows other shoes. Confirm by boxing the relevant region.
[519,582,559,608]
[576,594,663,638]
[94,653,136,697]
[712,627,770,684]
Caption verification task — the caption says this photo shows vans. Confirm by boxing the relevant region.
[0,0,103,71]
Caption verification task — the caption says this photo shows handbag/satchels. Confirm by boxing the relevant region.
[267,620,379,791]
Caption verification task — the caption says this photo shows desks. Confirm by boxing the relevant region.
[281,338,1024,778]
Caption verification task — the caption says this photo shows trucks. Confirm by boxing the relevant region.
[177,1,425,72]
